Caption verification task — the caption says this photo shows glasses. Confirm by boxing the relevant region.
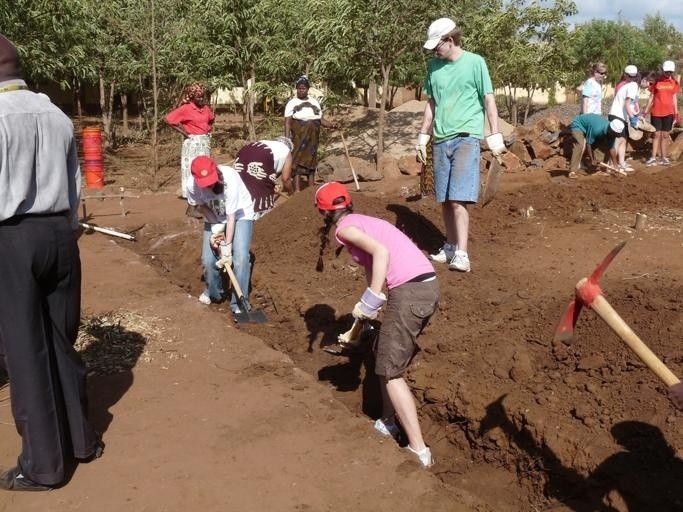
[597,70,606,75]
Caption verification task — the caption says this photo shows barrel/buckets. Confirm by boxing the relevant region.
[83,128,105,188]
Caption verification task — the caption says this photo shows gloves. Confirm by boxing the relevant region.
[210,223,234,272]
[606,155,671,175]
[673,114,680,127]
[352,287,387,321]
[486,132,507,165]
[415,134,431,166]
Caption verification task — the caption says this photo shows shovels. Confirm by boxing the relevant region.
[323,319,365,356]
[213,235,268,325]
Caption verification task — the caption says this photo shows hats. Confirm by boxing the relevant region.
[423,17,456,51]
[663,60,676,72]
[609,119,625,134]
[624,64,638,78]
[316,180,351,212]
[191,157,218,188]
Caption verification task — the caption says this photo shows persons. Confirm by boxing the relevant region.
[284,75,342,193]
[314,181,440,468]
[0,33,106,491]
[567,112,624,179]
[615,65,637,99]
[413,17,507,272]
[579,61,609,117]
[163,82,214,218]
[606,70,657,173]
[231,135,294,213]
[643,60,681,167]
[186,155,262,324]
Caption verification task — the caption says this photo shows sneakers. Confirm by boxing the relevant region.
[0,466,52,491]
[590,164,606,173]
[235,300,251,314]
[569,171,578,178]
[430,242,470,272]
[198,293,212,304]
[377,418,399,437]
[403,444,435,468]
